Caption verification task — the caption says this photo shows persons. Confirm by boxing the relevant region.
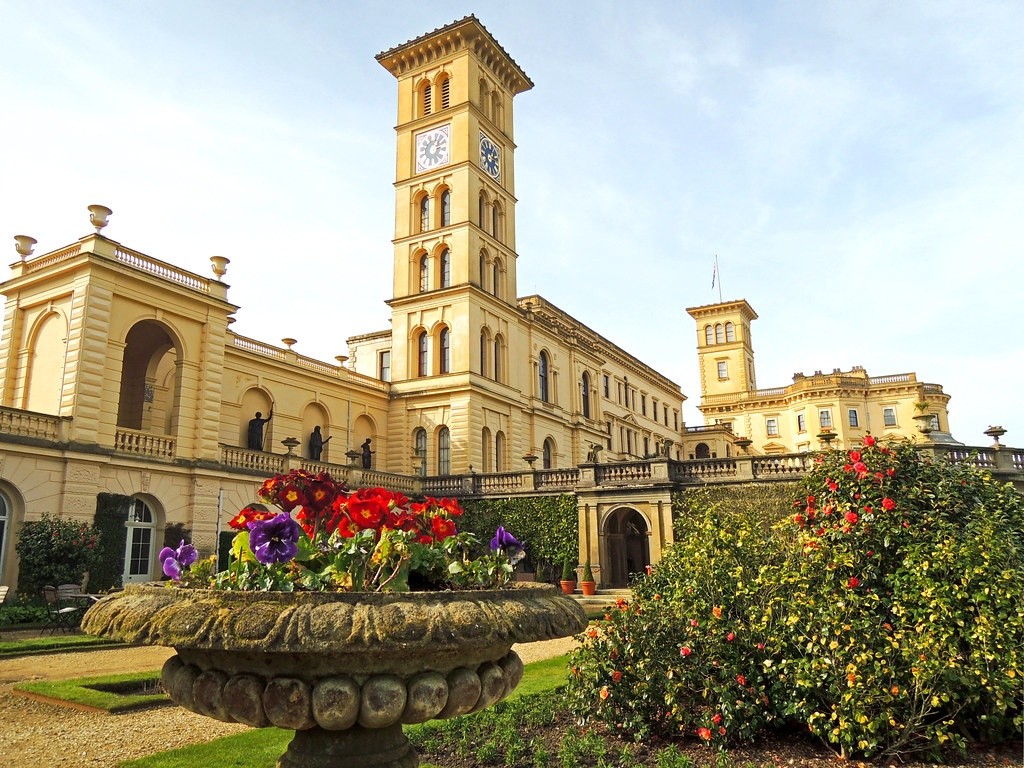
[247,410,272,450]
[309,426,323,461]
[361,438,375,469]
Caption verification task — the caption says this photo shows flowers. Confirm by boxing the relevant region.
[157,468,524,593]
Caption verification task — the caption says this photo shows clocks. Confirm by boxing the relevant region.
[417,130,448,169]
[480,137,500,179]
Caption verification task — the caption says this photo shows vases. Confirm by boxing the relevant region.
[77,578,588,768]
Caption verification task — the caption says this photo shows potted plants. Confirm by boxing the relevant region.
[560,556,577,596]
[581,558,597,595]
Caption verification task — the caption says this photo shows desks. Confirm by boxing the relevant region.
[69,594,105,632]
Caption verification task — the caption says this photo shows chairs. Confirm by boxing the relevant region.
[0,585,10,608]
[40,584,124,637]
